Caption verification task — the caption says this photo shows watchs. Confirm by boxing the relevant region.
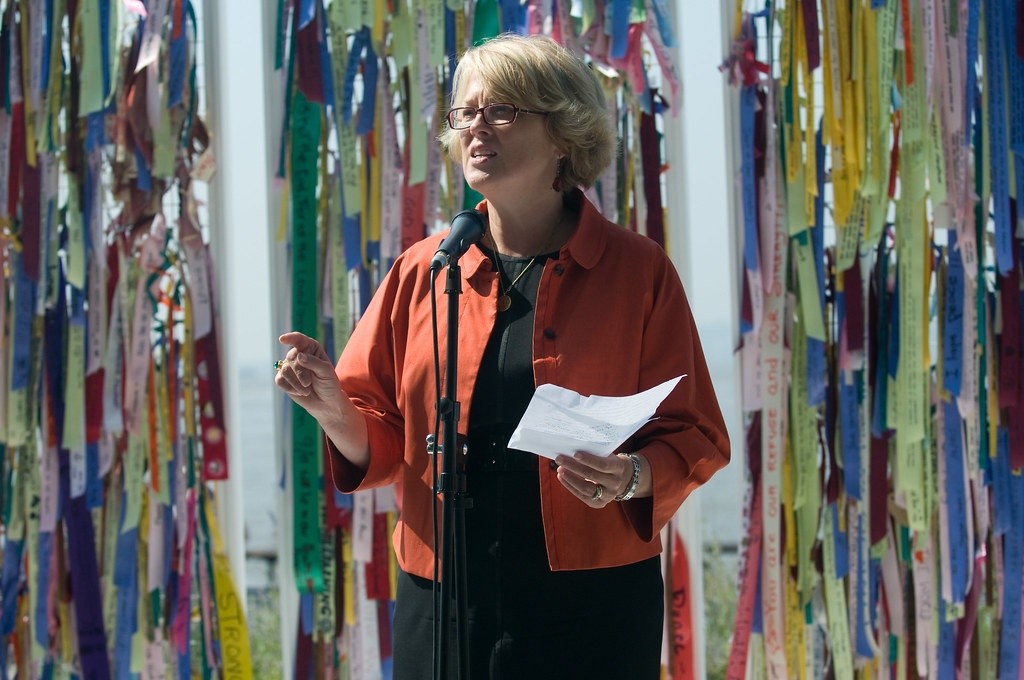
[614,452,641,502]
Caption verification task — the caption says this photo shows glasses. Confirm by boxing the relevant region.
[445,101,550,130]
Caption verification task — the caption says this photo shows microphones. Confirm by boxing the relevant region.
[429,209,486,272]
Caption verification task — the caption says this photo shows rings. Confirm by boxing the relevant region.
[592,483,602,503]
[274,360,285,370]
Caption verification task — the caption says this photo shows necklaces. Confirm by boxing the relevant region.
[484,213,566,312]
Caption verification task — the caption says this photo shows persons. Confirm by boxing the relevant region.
[273,33,729,680]
